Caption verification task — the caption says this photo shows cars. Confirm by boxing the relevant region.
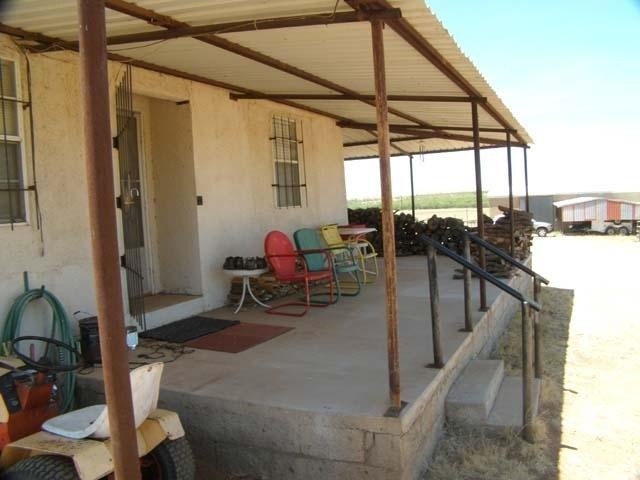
[491,208,554,238]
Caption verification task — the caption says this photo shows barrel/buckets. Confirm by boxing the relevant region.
[72,309,102,369]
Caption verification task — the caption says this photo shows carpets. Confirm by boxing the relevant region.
[137,315,241,344]
[182,322,301,356]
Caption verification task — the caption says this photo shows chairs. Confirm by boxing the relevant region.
[318,222,380,297]
[291,226,361,307]
[40,359,165,442]
[262,230,337,318]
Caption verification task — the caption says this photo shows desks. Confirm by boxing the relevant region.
[337,225,384,282]
[223,265,273,314]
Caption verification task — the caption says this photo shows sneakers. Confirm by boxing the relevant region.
[245,256,266,269]
[223,256,243,269]
